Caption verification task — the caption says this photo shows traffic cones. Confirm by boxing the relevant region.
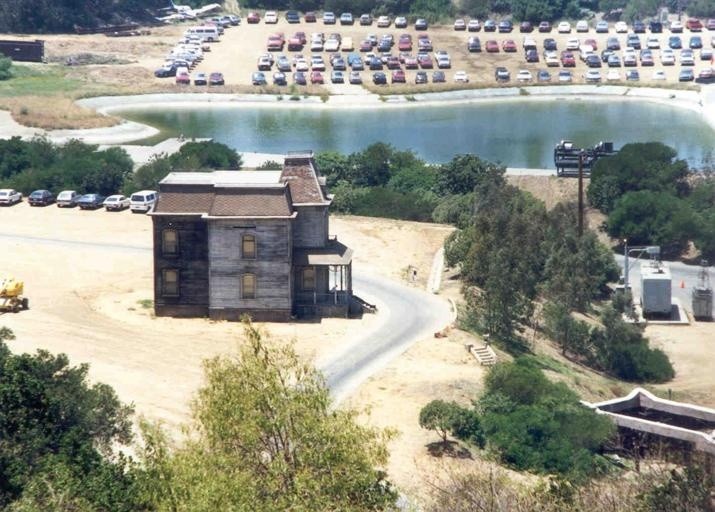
[679,280,686,288]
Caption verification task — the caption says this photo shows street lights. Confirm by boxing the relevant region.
[623,239,662,316]
[553,139,620,238]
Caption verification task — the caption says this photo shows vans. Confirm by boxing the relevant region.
[128,190,158,213]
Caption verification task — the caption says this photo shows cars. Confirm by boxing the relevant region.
[0,189,23,206]
[193,73,207,85]
[76,195,106,209]
[154,14,241,77]
[252,72,267,86]
[468,36,481,52]
[454,19,466,31]
[176,71,191,84]
[57,191,81,207]
[208,72,225,85]
[27,189,56,207]
[258,9,451,86]
[469,19,481,30]
[247,12,261,24]
[483,18,715,86]
[103,194,130,211]
[452,70,469,83]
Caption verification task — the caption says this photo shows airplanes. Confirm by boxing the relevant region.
[154,1,222,25]
